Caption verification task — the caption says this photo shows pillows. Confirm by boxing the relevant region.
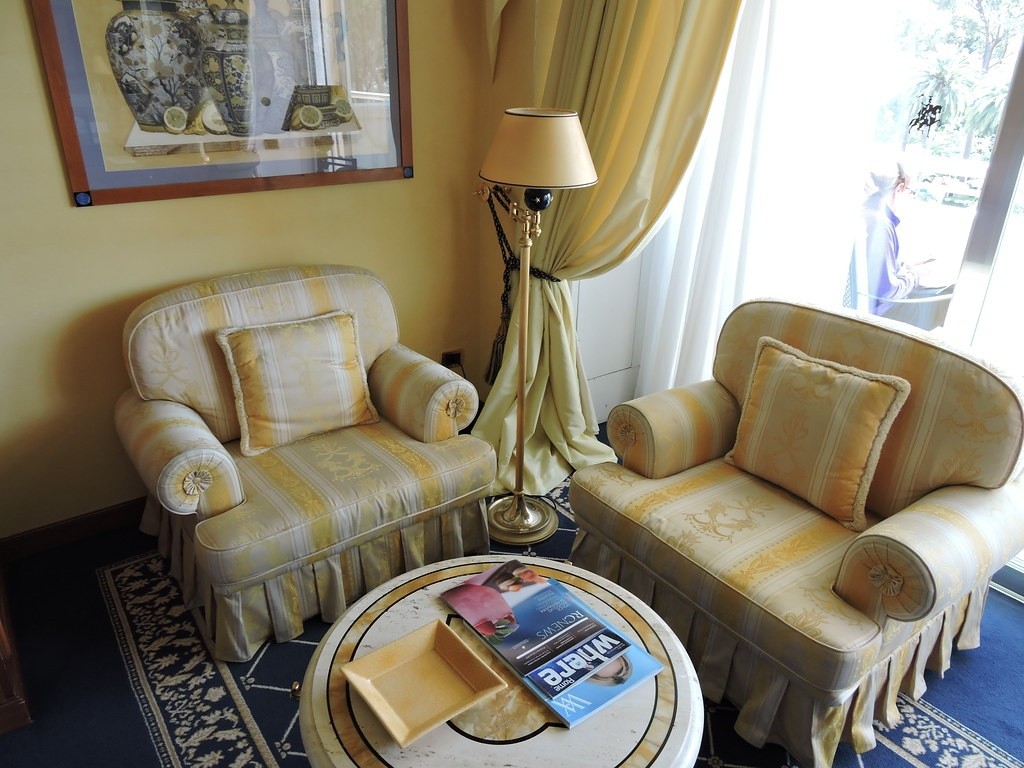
[722,335,910,532]
[214,309,380,458]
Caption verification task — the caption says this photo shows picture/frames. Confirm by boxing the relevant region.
[32,0,414,207]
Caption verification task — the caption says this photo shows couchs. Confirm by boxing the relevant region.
[113,264,497,662]
[569,297,1024,768]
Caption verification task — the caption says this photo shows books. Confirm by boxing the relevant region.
[440,558,664,729]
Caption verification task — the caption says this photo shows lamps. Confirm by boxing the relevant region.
[281,84,362,172]
[479,107,600,545]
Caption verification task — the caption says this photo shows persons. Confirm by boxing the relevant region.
[587,656,633,691]
[845,160,935,317]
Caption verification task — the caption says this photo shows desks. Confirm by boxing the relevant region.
[292,555,705,768]
